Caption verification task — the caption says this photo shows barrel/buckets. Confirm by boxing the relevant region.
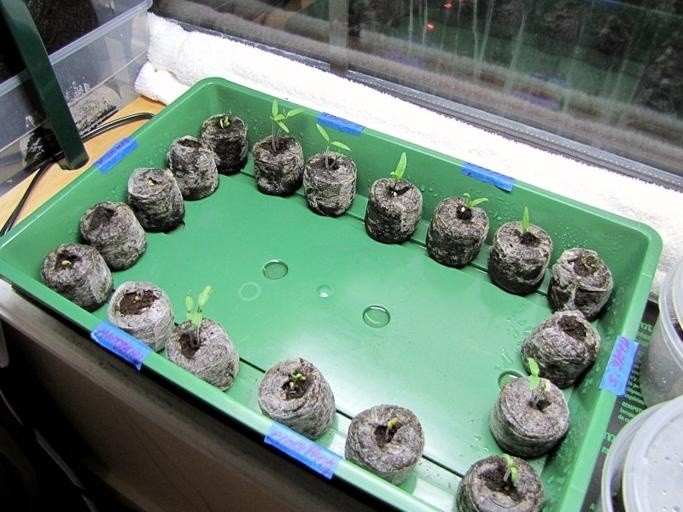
[639,256,683,408]
[600,393,682,511]
